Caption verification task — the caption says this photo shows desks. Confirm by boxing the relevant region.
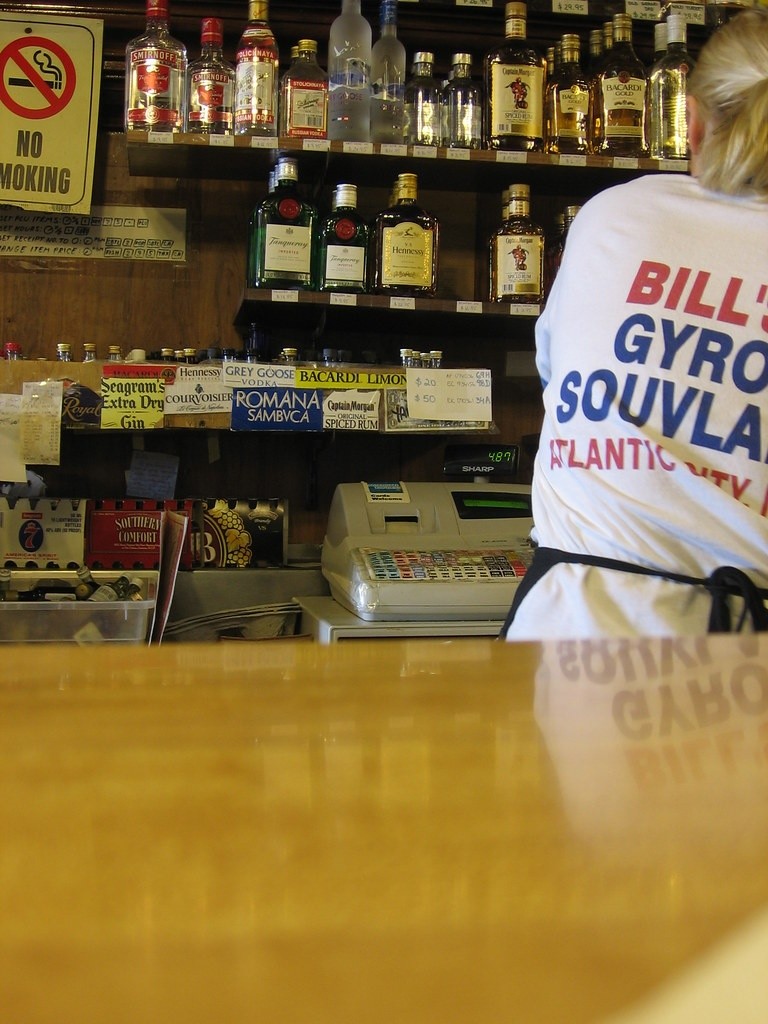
[1,630,767,1024]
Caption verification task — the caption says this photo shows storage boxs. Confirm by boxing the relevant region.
[0,572,158,644]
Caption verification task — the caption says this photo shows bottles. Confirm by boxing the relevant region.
[184,18,236,135]
[234,0,277,137]
[278,39,328,139]
[247,156,440,299]
[371,0,406,144]
[0,322,260,364]
[488,183,582,302]
[408,2,698,159]
[124,0,189,132]
[328,0,372,142]
[279,346,444,370]
[0,567,143,602]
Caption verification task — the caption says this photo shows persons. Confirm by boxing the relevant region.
[500,12,768,638]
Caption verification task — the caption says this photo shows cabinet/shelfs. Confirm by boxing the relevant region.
[0,1,753,570]
